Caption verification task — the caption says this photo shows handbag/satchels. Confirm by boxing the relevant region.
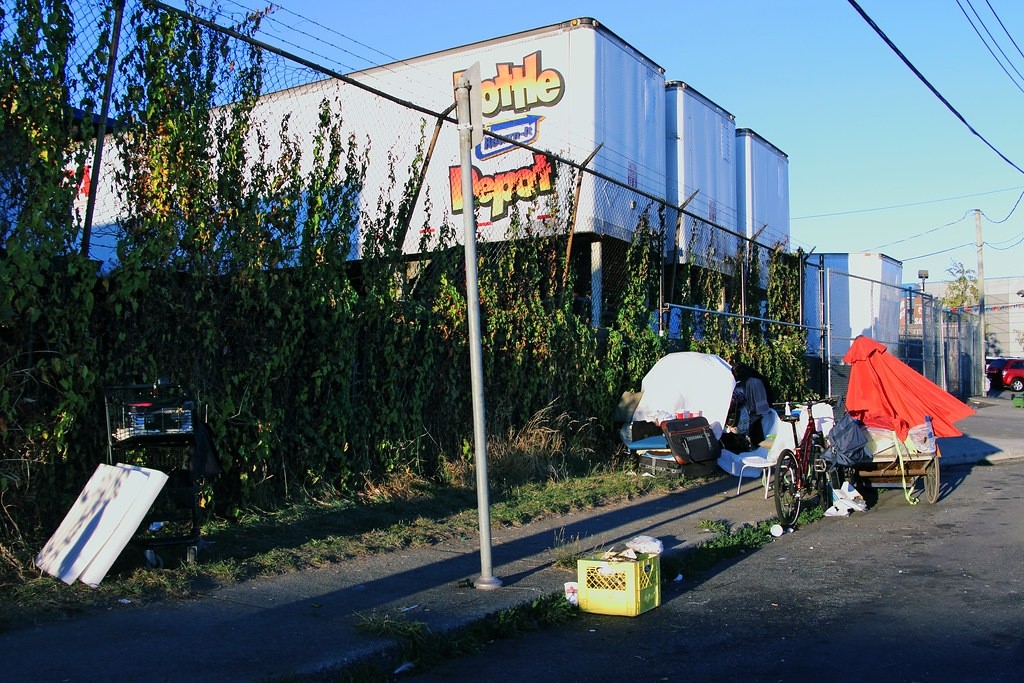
[821,412,869,465]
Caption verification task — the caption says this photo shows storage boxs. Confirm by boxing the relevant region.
[631,414,720,480]
[572,551,662,618]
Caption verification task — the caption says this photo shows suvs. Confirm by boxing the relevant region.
[985,355,1024,392]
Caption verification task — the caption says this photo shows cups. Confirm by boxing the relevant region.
[676,410,685,419]
[690,411,702,418]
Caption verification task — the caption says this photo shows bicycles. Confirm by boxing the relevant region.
[773,398,832,527]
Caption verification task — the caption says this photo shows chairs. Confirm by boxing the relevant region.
[737,429,798,500]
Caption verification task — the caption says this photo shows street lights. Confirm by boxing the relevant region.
[918,269,929,293]
